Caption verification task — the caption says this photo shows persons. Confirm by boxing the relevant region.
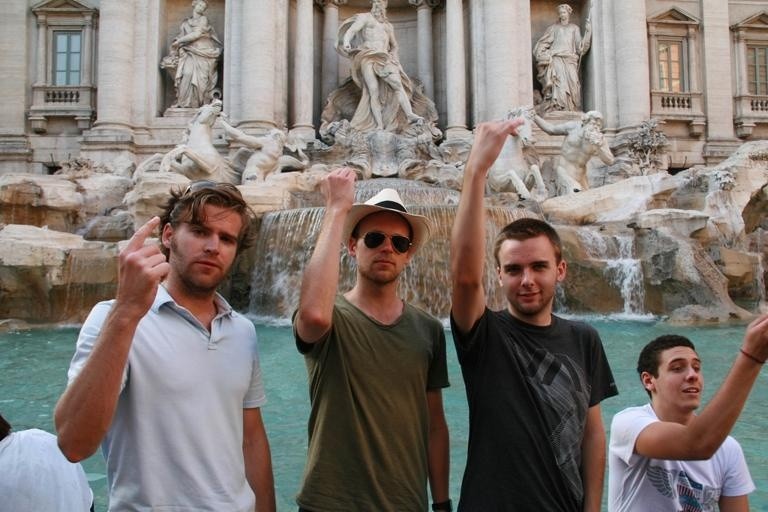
[342,0,423,130]
[219,107,284,189]
[172,0,224,108]
[527,106,616,193]
[289,168,451,512]
[534,3,592,113]
[54,182,278,512]
[0,411,95,512]
[609,309,767,512]
[451,117,620,511]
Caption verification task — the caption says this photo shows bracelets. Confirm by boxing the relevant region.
[431,499,450,510]
[740,348,765,364]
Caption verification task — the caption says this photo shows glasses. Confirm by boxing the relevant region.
[359,231,414,255]
[167,181,217,217]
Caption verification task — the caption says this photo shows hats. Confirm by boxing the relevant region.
[340,189,432,258]
